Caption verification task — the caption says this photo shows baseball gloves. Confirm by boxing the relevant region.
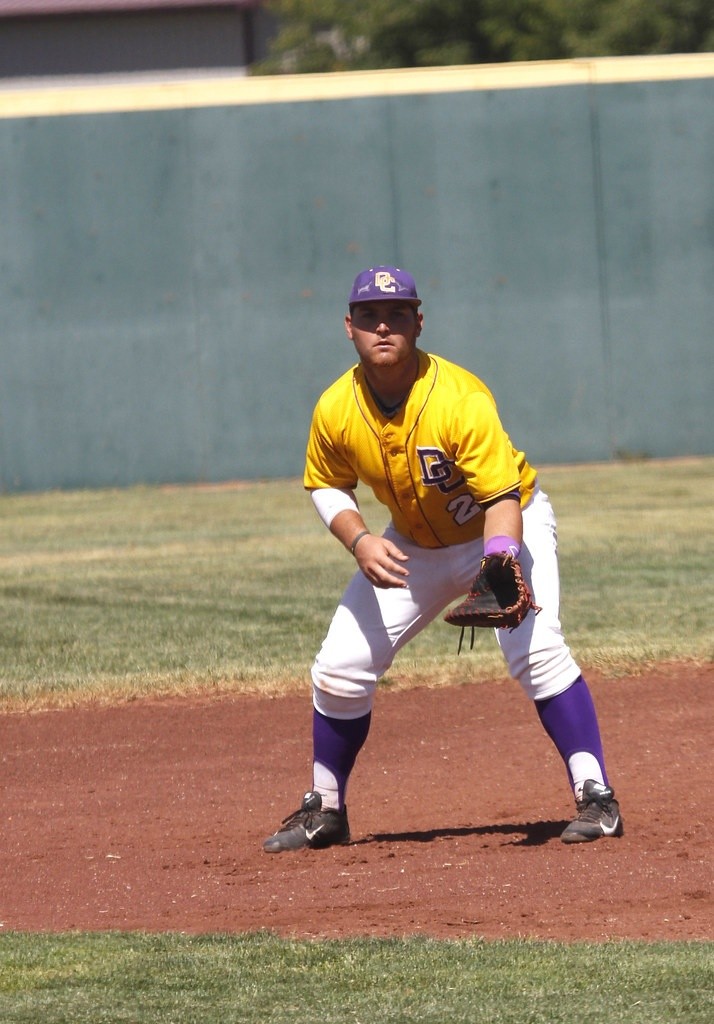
[444,550,544,657]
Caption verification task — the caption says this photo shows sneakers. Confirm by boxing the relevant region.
[263,790,351,853]
[561,778,624,842]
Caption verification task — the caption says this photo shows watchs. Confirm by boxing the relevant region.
[351,531,370,555]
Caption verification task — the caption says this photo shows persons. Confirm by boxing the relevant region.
[264,265,622,854]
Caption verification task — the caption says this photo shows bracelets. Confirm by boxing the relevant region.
[484,536,521,560]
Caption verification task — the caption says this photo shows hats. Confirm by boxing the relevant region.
[348,267,422,308]
